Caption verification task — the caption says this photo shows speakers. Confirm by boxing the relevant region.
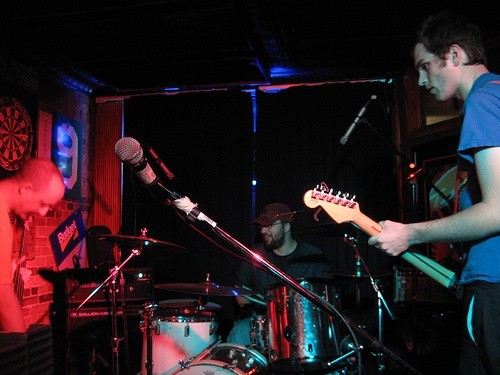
[384,301,464,375]
[51,303,153,375]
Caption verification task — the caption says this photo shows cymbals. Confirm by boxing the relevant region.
[151,299,222,308]
[155,283,259,296]
[94,234,188,252]
[333,272,384,278]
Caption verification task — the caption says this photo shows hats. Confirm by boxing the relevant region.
[252,203,294,226]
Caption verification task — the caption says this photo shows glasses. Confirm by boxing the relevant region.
[257,222,284,229]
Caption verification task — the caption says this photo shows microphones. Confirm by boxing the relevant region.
[340,95,375,145]
[115,137,168,199]
[149,148,174,180]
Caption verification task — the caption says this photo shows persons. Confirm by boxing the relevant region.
[368,9,500,375]
[0,158,66,333]
[233,203,328,375]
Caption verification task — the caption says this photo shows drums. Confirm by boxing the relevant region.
[151,310,218,375]
[164,343,268,375]
[266,278,341,367]
[249,318,268,354]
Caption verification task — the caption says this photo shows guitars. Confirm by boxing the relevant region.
[303,185,478,347]
